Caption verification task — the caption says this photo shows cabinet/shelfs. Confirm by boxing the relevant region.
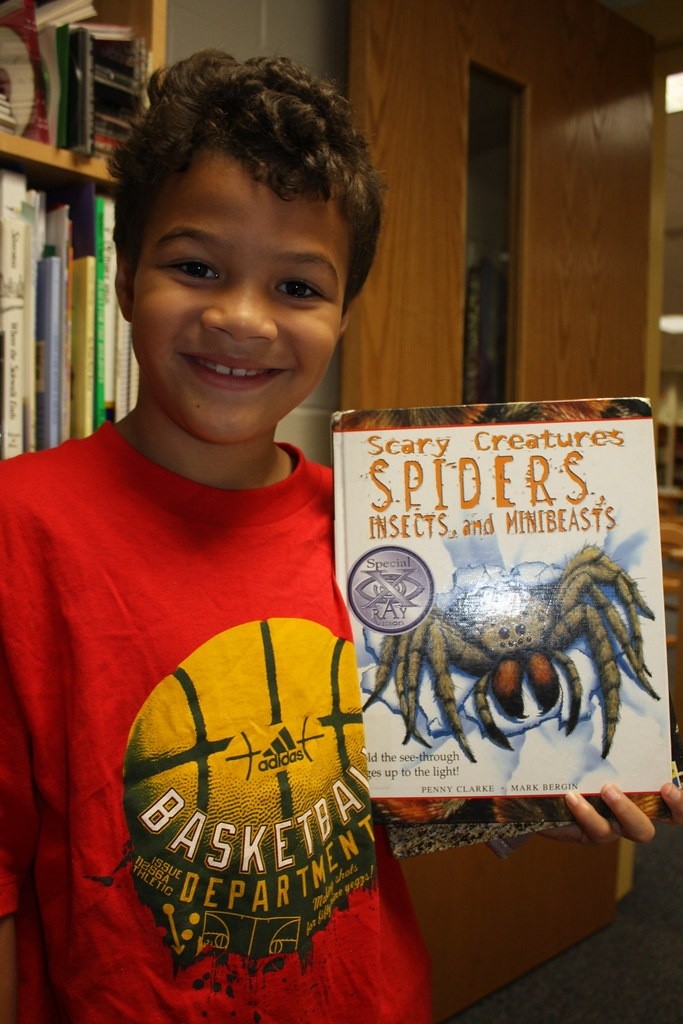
[0,0,167,189]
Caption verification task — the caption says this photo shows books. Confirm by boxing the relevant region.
[0,0,140,157]
[0,169,139,465]
[329,397,681,858]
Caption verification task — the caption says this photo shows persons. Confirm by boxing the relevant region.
[0,45,683,1024]
